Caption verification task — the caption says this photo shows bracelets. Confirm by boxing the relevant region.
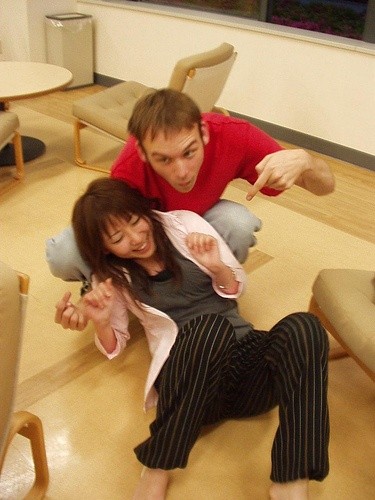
[215,266,238,291]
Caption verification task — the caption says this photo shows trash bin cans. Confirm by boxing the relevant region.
[45,12,95,90]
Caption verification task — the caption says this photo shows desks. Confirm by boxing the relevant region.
[0,61,74,180]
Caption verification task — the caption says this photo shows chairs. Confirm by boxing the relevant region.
[0,261,50,499]
[307,267,375,381]
[0,111,24,181]
[72,41,238,174]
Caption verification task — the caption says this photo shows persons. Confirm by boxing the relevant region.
[74,175,330,500]
[45,88,335,332]
